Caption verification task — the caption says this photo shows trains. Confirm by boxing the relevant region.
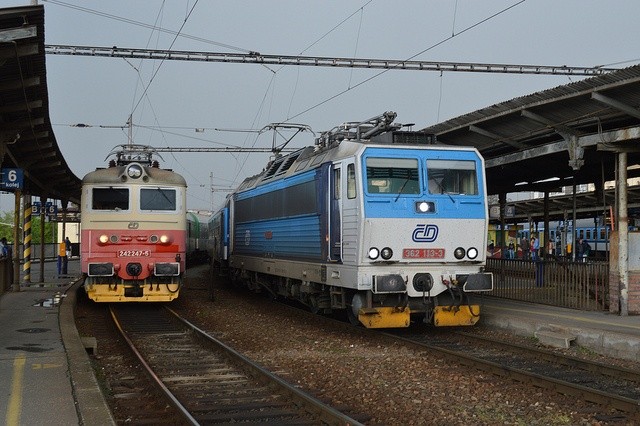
[81,143,210,304]
[207,111,493,329]
[516,226,639,258]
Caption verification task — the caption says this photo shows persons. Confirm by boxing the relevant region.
[530,237,535,265]
[65,237,71,260]
[0,237,14,285]
[508,240,515,259]
[533,235,541,261]
[576,239,583,262]
[489,239,495,258]
